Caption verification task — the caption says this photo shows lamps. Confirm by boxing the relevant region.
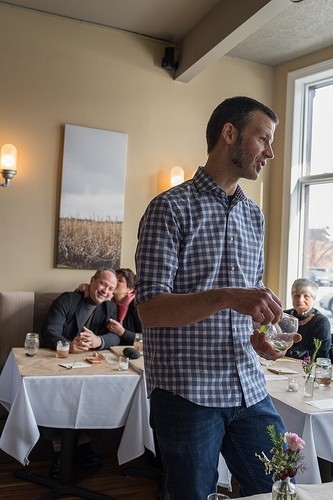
[170,166,184,187]
[0,144,17,187]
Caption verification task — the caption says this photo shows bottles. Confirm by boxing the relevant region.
[315,358,333,387]
[134,333,143,356]
[24,333,39,359]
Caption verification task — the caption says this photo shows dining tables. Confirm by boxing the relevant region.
[0,347,157,486]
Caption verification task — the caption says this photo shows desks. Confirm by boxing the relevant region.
[218,355,333,492]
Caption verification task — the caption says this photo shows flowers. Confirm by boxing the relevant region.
[300,337,323,374]
[254,424,308,483]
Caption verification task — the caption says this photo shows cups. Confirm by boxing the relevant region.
[57,340,70,358]
[207,493,231,500]
[288,376,299,392]
[259,312,298,351]
[119,357,129,371]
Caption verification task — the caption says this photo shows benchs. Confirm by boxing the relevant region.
[0,290,61,375]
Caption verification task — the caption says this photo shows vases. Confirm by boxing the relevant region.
[304,370,315,398]
[272,477,298,500]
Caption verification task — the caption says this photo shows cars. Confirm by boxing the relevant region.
[313,277,333,313]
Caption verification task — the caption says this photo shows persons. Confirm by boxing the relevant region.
[134,96,302,500]
[74,268,142,346]
[39,266,120,453]
[278,279,332,362]
